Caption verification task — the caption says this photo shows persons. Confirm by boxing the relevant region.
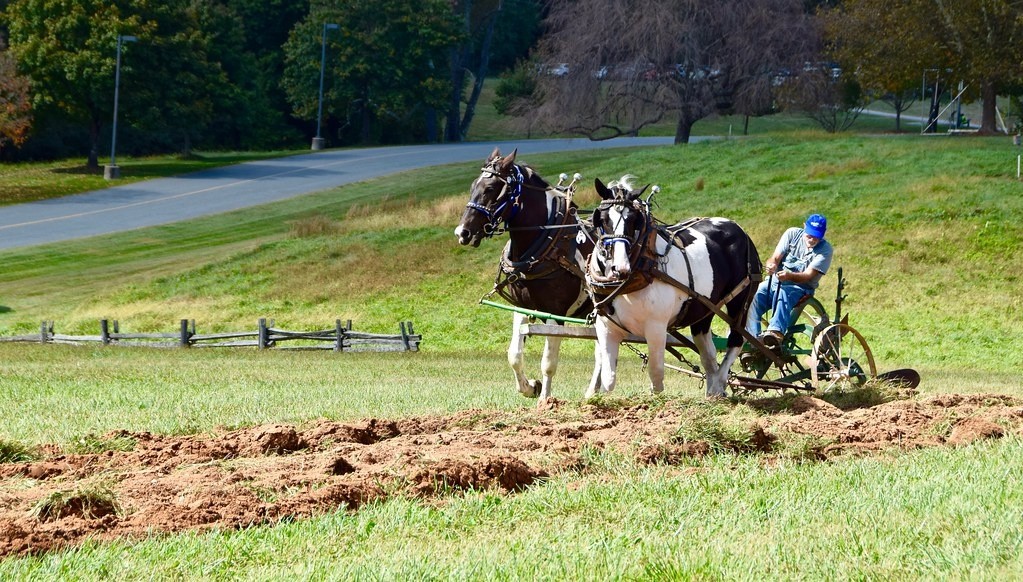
[741,215,834,364]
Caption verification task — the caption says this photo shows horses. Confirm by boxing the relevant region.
[453,147,764,409]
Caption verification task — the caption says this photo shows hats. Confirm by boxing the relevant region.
[804,214,826,240]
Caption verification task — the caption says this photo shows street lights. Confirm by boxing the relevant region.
[109,35,139,165]
[317,23,338,138]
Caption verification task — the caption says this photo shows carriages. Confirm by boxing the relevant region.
[454,146,920,409]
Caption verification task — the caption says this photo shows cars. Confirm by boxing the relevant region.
[529,63,719,80]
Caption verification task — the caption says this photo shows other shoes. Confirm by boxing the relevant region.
[763,331,783,355]
[741,352,757,362]
